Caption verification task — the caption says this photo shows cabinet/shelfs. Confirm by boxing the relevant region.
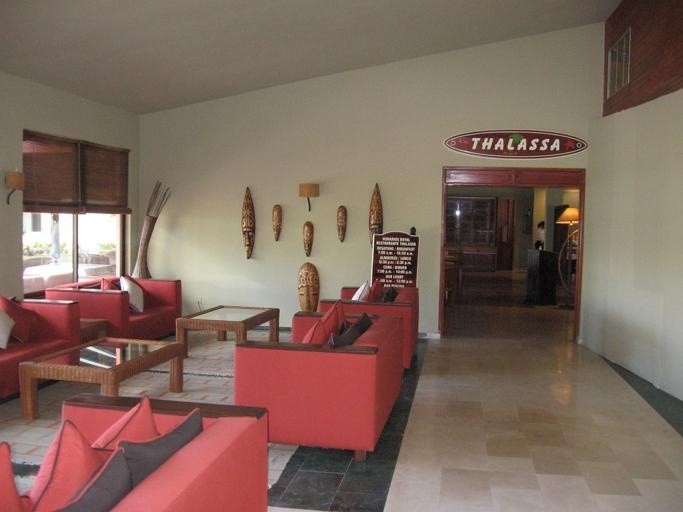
[527,248,558,305]
[447,196,498,247]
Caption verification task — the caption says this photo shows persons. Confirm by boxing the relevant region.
[535,221,544,250]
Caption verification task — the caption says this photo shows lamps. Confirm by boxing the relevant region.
[299,183,320,213]
[5,172,24,207]
[556,207,579,251]
